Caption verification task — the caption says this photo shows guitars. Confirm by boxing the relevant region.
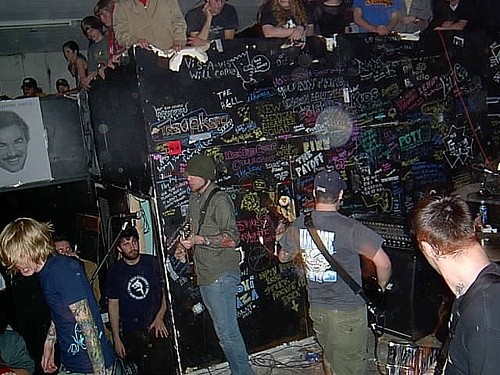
[275,207,385,323]
[181,222,197,289]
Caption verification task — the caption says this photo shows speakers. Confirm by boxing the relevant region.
[359,245,416,336]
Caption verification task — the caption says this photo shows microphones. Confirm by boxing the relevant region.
[113,210,142,219]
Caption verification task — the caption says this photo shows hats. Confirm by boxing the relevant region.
[314,170,347,194]
[180,156,217,180]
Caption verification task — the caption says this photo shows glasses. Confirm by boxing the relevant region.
[57,247,72,254]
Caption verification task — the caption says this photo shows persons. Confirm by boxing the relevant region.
[101,223,173,375]
[171,147,258,375]
[0,217,108,375]
[0,0,500,102]
[410,189,500,375]
[0,109,31,175]
[275,162,401,375]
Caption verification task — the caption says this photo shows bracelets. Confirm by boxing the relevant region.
[298,24,308,33]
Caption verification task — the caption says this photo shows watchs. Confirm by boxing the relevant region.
[412,15,421,25]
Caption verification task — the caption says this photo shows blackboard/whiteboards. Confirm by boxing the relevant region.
[134,25,500,373]
